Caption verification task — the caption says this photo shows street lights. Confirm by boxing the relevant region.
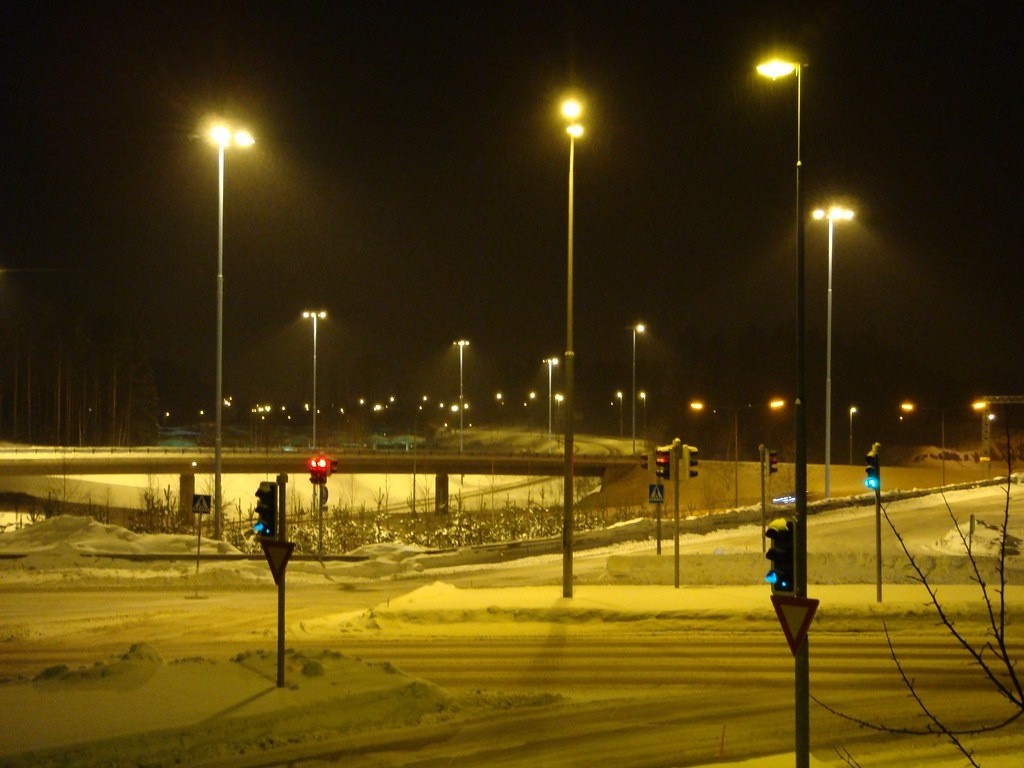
[617,392,624,438]
[204,122,258,542]
[849,406,857,465]
[811,206,856,498]
[560,98,584,597]
[453,339,470,453]
[689,397,788,511]
[899,400,991,486]
[757,53,811,768]
[303,311,327,448]
[632,324,645,455]
[542,358,559,455]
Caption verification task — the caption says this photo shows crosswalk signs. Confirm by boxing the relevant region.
[648,484,664,503]
[192,494,212,514]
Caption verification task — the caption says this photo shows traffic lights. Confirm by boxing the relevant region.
[255,481,278,539]
[683,445,699,480]
[765,448,777,477]
[641,451,653,470]
[765,516,799,598]
[656,444,674,480]
[308,452,328,484]
[865,450,880,489]
